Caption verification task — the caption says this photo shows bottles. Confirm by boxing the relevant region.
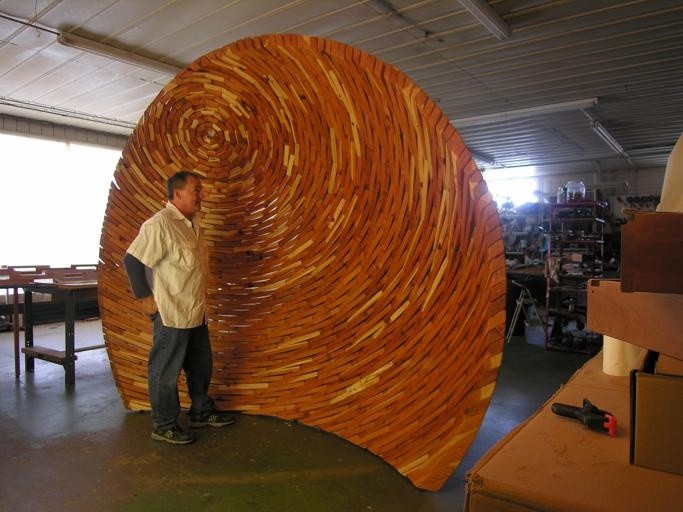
[557,180,585,203]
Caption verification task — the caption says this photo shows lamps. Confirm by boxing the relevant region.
[55,31,183,78]
[456,0,511,41]
[469,151,496,166]
[591,121,623,154]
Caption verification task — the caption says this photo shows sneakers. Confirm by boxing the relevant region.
[150,423,195,444]
[188,409,236,428]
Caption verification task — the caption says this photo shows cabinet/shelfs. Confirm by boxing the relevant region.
[500,202,606,356]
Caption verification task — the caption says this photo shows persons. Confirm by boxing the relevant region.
[524,232,546,265]
[123,171,235,445]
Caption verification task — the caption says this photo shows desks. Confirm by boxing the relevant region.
[21,279,105,385]
